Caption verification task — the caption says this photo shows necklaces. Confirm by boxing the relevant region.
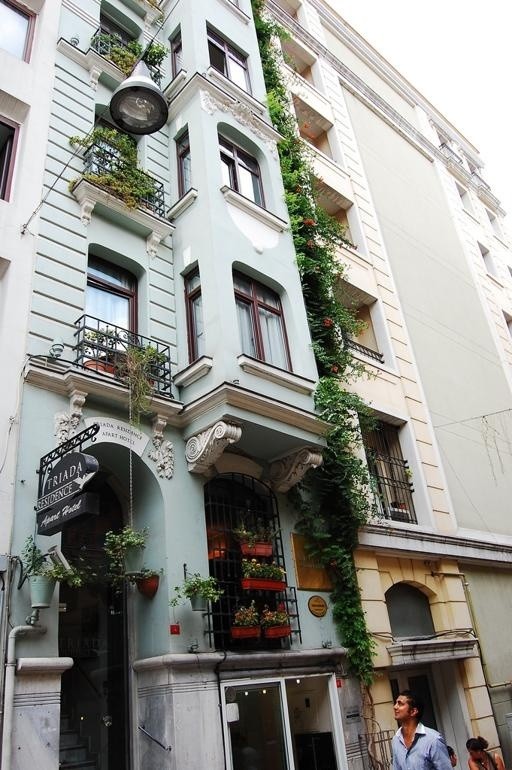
[480,758,486,765]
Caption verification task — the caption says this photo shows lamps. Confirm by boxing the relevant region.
[110,61,169,134]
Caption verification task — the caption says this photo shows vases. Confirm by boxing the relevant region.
[191,595,208,611]
[233,626,291,639]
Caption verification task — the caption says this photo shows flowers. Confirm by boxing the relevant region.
[230,522,290,627]
[170,574,225,606]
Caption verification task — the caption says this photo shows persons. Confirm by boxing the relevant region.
[466,736,505,770]
[446,745,457,767]
[392,689,455,770]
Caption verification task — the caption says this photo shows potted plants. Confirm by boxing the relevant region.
[85,329,169,427]
